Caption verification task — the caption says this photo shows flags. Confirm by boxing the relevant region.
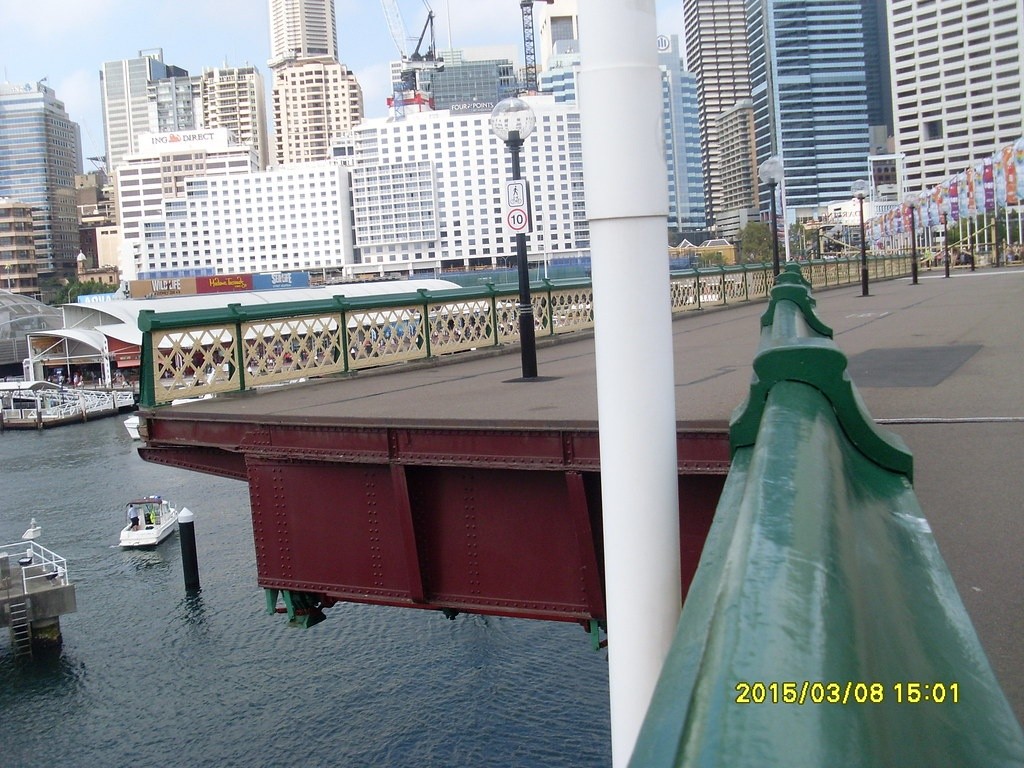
[864,135,1024,244]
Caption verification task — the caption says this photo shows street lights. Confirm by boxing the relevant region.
[851,179,870,218]
[760,159,787,213]
[939,204,951,233]
[966,208,976,237]
[489,99,537,177]
[905,196,918,235]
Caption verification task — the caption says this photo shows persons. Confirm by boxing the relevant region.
[128,504,140,528]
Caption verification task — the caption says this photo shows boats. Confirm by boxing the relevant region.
[117,496,178,546]
